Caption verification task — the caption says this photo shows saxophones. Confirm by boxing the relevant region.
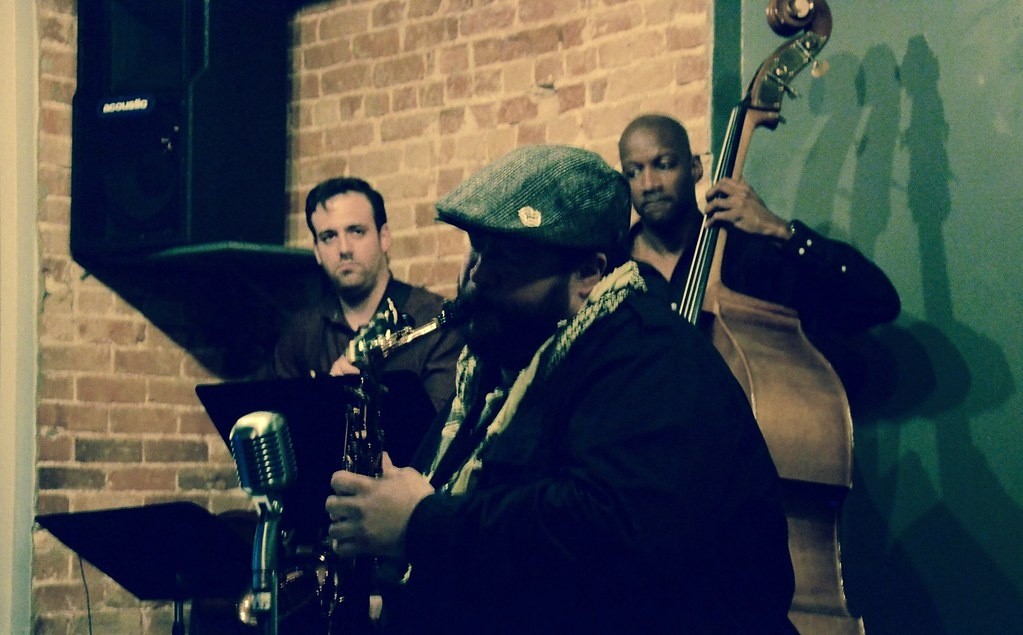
[315,299,470,635]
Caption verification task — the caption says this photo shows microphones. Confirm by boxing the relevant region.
[230,409,297,495]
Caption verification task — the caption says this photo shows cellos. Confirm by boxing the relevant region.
[676,0,868,635]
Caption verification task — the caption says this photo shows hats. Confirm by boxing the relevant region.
[433,144,633,249]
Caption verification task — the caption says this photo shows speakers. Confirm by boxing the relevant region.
[70,1,289,271]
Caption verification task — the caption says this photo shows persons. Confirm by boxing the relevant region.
[615,113,905,354]
[256,176,463,423]
[327,146,799,635]
